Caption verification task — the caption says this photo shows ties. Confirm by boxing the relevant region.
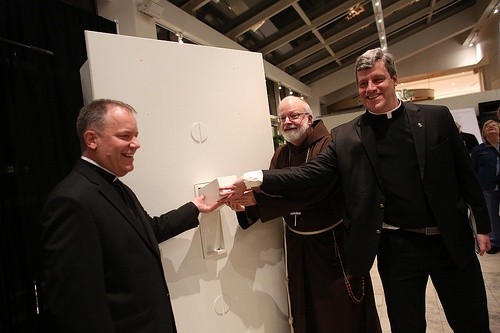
[115,178,152,244]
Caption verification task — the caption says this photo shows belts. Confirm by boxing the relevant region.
[397,226,441,234]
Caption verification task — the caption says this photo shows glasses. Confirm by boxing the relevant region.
[277,113,307,122]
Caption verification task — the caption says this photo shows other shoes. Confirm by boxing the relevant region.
[486,245,499,254]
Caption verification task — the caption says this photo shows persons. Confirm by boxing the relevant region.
[36,98,226,333]
[471,120,500,254]
[219,95,382,333]
[218,47,492,333]
[454,121,479,152]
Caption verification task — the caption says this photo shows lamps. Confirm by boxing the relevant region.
[277,82,304,99]
[371,0,388,53]
